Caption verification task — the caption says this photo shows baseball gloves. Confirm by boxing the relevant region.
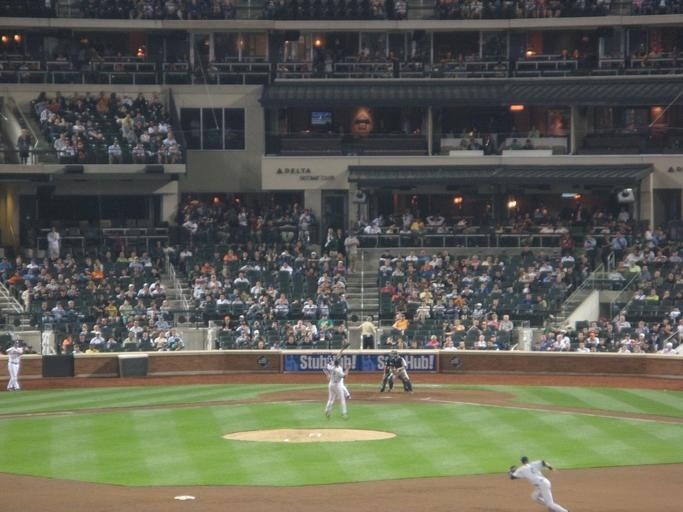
[508,466,516,478]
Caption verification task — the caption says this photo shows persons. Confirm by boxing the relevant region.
[377,355,410,394]
[0,202,358,355]
[1,1,683,19]
[507,455,568,511]
[2,337,26,393]
[2,36,683,84]
[617,123,680,150]
[30,91,183,164]
[386,350,415,393]
[353,203,683,355]
[296,120,344,150]
[321,356,353,402]
[16,129,31,164]
[412,116,541,154]
[323,359,352,420]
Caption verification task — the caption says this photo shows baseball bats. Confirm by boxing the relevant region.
[336,342,350,360]
